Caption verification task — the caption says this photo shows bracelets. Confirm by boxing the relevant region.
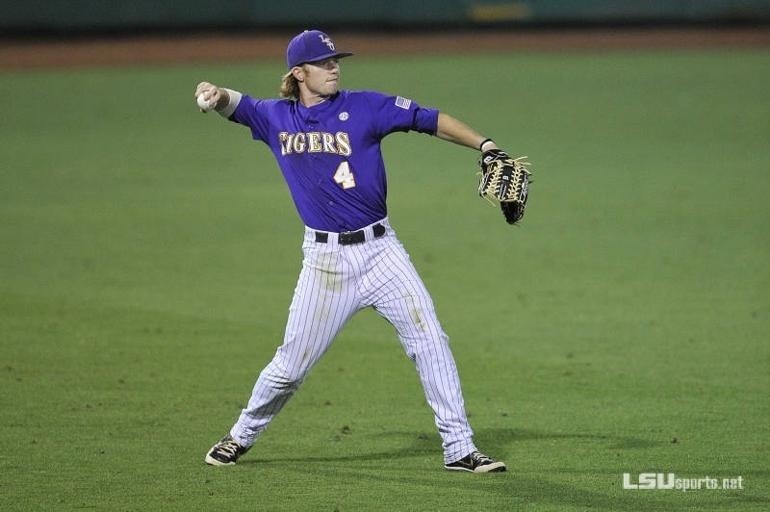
[478,138,496,152]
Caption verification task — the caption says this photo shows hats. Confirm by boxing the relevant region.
[286,29,354,68]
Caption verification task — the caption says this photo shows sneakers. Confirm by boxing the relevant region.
[444,450,507,475]
[205,432,253,467]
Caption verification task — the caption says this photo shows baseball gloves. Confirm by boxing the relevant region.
[475,149,535,224]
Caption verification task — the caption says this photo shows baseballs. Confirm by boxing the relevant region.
[196,91,217,112]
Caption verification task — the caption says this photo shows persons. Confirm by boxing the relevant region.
[193,28,535,475]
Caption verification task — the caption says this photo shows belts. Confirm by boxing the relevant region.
[314,223,386,246]
